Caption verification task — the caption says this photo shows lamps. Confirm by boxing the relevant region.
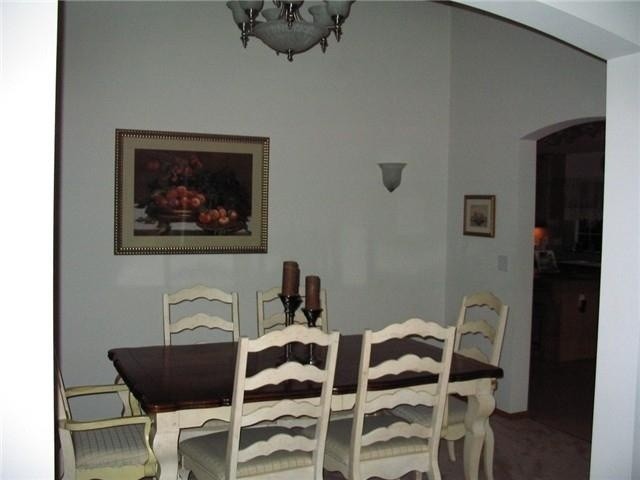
[377,161,407,192]
[222,0,353,65]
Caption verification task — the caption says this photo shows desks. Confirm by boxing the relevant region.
[108,333,504,479]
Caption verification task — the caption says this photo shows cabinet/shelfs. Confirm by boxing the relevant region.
[537,269,599,369]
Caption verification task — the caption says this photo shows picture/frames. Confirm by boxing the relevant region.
[462,191,496,237]
[114,128,270,256]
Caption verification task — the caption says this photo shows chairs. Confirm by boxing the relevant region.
[384,289,510,479]
[55,349,159,480]
[302,317,456,480]
[256,284,329,337]
[178,325,340,479]
[162,284,240,345]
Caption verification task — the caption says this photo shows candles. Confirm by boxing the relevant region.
[306,274,321,311]
[280,260,301,296]
